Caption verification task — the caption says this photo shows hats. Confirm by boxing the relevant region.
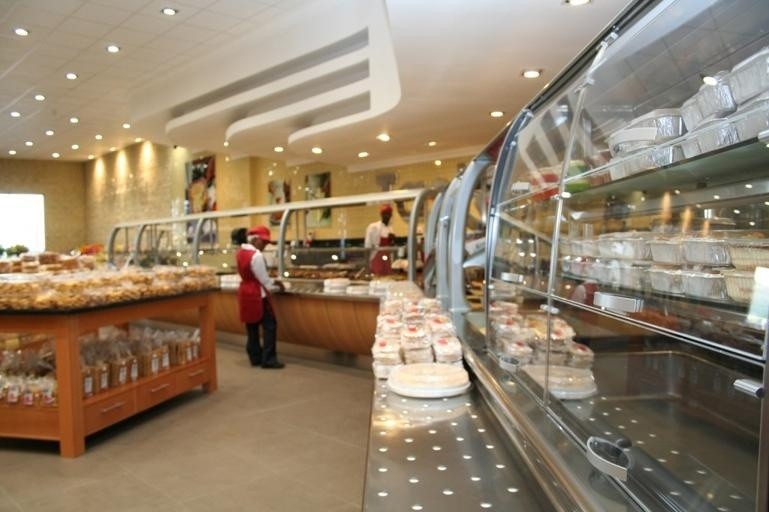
[247,226,271,242]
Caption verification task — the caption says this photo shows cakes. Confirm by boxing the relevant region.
[322,277,468,400]
[488,297,597,399]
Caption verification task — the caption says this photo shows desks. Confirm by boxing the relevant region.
[1,289,221,458]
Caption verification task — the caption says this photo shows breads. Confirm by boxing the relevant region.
[0,249,220,407]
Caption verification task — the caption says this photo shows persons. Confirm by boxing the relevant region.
[364,203,396,276]
[235,225,284,368]
[567,258,600,345]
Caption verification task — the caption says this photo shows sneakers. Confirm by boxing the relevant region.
[250,358,285,368]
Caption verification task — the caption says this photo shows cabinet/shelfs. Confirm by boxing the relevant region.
[107,193,418,298]
[422,0,769,511]
